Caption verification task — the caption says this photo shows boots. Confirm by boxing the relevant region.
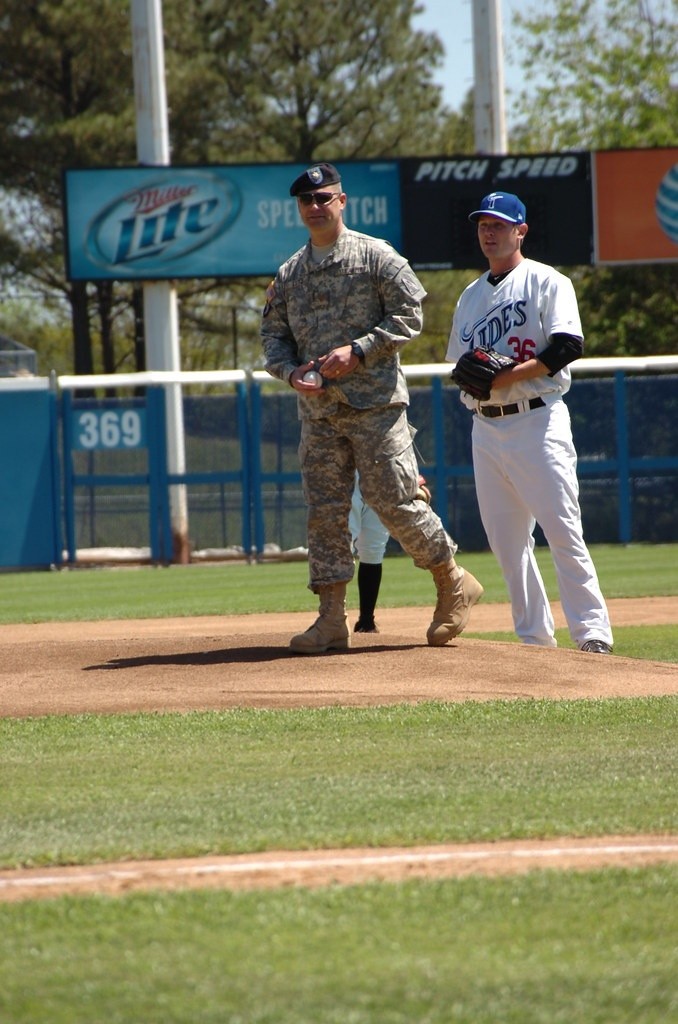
[289,580,348,653]
[427,556,486,645]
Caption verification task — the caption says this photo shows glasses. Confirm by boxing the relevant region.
[297,192,340,205]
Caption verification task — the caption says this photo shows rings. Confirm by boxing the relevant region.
[335,370,339,374]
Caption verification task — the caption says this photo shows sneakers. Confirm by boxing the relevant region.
[582,640,614,654]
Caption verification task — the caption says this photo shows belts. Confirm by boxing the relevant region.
[474,396,545,418]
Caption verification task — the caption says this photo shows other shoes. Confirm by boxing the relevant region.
[354,623,378,633]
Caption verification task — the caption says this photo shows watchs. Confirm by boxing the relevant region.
[352,343,364,361]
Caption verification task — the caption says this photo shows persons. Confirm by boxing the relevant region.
[444,191,615,655]
[260,162,484,654]
[346,467,432,633]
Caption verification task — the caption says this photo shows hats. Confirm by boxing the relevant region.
[289,163,339,194]
[469,192,526,223]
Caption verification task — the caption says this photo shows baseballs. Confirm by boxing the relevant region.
[303,371,325,387]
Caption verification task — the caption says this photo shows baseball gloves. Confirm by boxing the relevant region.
[450,347,522,400]
